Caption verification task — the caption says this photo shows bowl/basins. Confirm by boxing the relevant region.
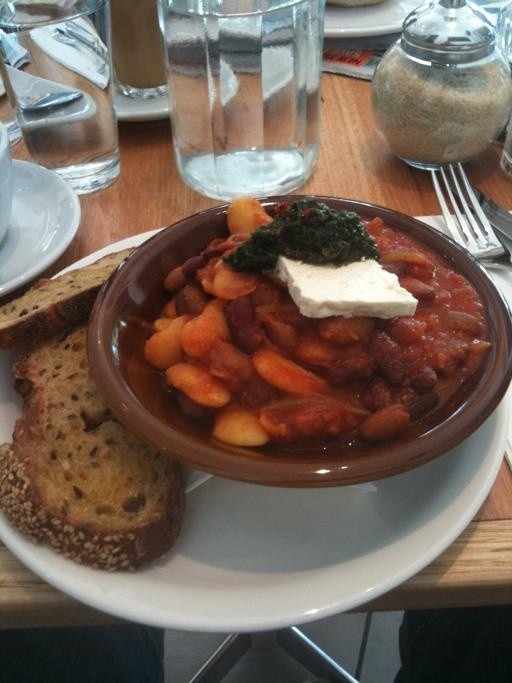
[90,194,512,490]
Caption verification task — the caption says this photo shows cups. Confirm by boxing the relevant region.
[325,2,427,41]
[1,0,123,197]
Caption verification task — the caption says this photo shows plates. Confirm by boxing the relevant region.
[1,155,81,294]
[0,224,512,633]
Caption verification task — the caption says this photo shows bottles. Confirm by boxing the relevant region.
[171,0,326,202]
[104,0,173,99]
[369,1,512,173]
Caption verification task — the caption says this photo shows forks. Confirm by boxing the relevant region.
[430,164,506,273]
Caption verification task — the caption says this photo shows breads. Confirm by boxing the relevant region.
[0,319,186,573]
[0,247,137,350]
